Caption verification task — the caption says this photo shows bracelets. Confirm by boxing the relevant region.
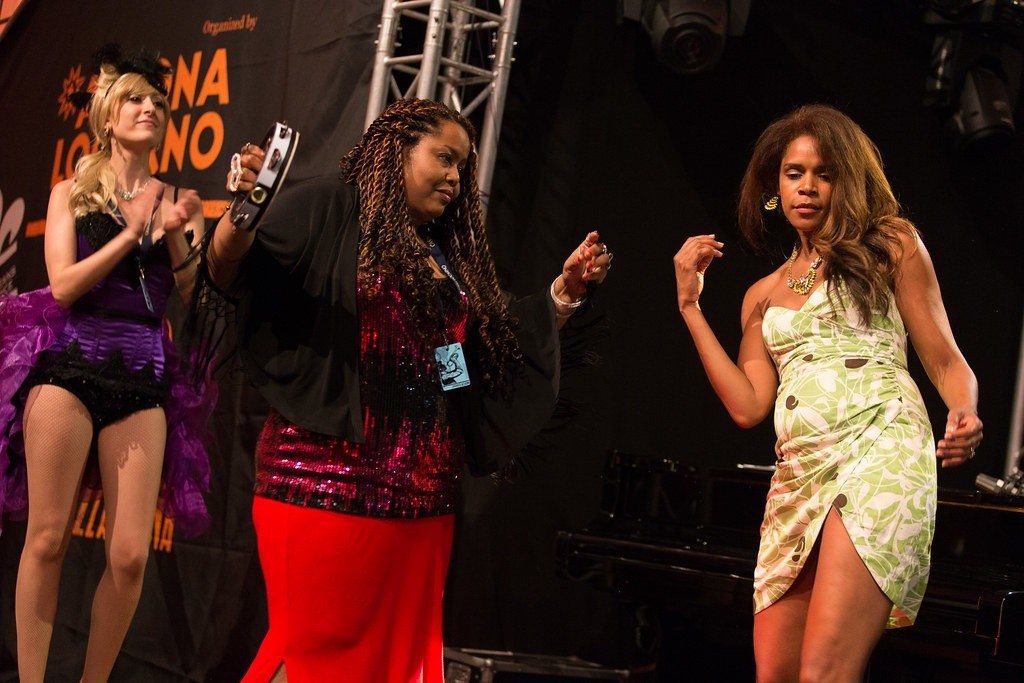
[551,274,582,308]
[556,309,575,319]
[170,253,197,270]
[173,270,198,290]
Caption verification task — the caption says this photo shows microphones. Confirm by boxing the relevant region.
[975,474,1020,496]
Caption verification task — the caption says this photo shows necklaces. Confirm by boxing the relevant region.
[115,175,153,201]
[787,244,822,294]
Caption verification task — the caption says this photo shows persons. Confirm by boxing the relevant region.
[200,99,614,683]
[15,65,203,683]
[673,108,983,683]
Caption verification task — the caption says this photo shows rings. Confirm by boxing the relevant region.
[970,447,976,458]
[602,242,613,269]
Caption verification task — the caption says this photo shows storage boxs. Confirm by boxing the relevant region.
[441,644,630,683]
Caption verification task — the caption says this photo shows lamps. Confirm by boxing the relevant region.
[920,30,966,113]
[948,46,1015,147]
